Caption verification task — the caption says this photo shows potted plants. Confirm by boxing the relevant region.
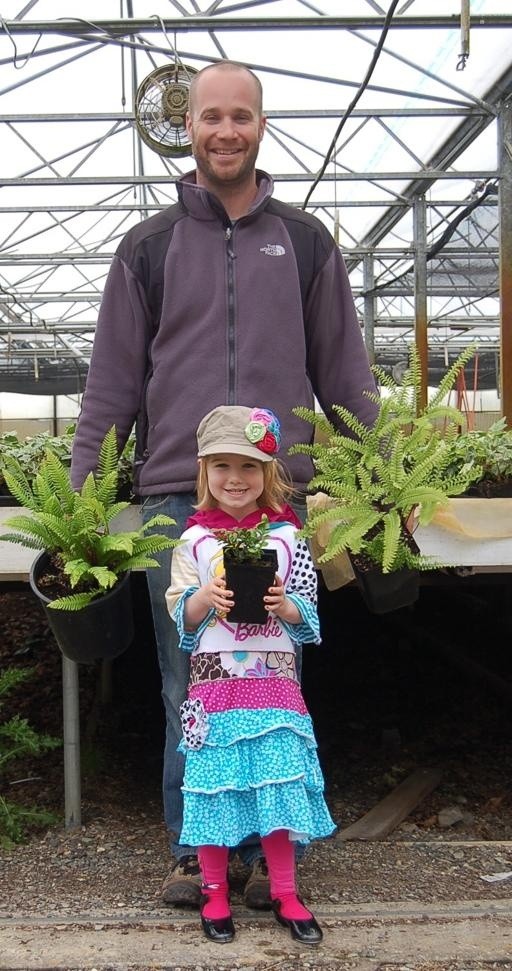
[0,421,188,673]
[284,337,512,614]
[210,512,278,628]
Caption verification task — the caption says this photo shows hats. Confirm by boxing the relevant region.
[194,404,281,464]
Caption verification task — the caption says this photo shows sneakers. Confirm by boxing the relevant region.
[161,854,206,907]
[198,891,236,944]
[243,856,275,912]
[272,893,324,945]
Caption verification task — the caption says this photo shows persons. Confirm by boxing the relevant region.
[68,60,416,912]
[164,404,338,947]
